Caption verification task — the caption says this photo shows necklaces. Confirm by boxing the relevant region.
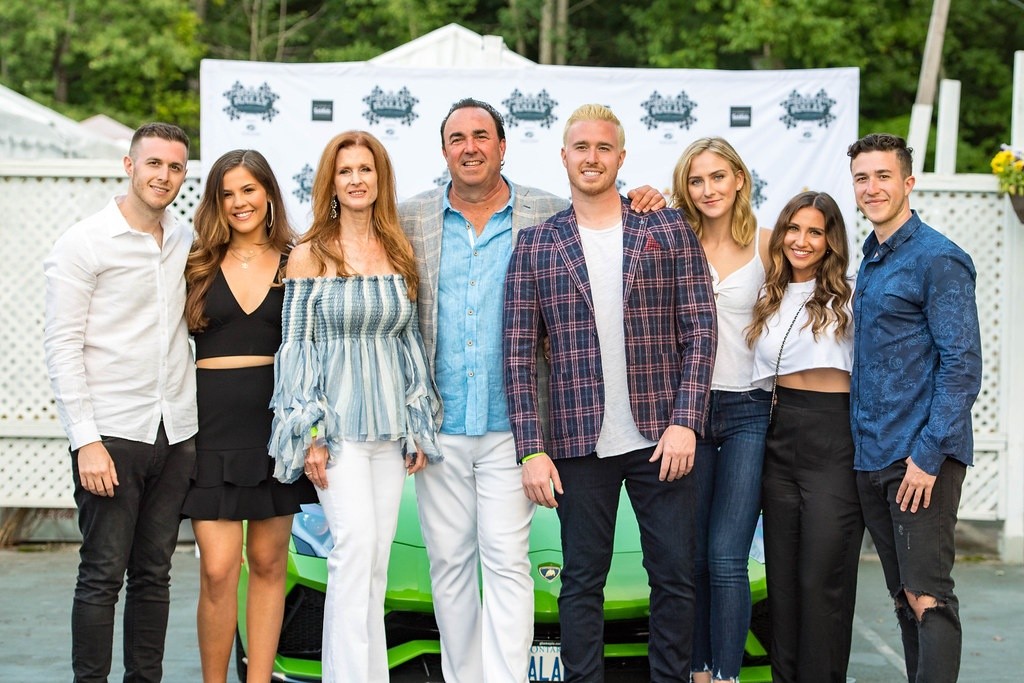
[226,245,272,269]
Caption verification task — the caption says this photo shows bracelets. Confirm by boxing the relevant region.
[516,452,545,464]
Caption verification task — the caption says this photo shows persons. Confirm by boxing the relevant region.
[181,147,320,683]
[42,119,199,683]
[500,99,720,683]
[750,193,868,683]
[388,99,669,683]
[669,136,774,683]
[844,133,984,683]
[265,131,446,683]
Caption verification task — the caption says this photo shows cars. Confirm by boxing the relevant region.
[233,467,773,683]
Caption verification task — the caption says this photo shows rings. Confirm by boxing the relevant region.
[304,471,312,477]
